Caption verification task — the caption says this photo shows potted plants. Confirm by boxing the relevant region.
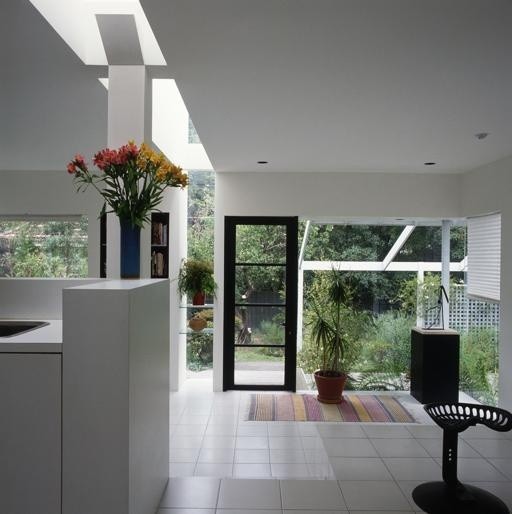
[304,263,359,404]
[170,257,219,305]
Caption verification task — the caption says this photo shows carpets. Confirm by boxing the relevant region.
[244,395,421,423]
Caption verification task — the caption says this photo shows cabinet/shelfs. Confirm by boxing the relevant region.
[179,305,214,335]
[0,352,62,514]
[100,212,169,278]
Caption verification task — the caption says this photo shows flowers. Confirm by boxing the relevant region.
[67,139,189,231]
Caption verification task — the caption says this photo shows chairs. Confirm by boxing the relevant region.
[424,402,512,514]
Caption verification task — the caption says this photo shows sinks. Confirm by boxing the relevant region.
[0,320,50,338]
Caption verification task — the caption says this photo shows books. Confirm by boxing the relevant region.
[152,220,168,276]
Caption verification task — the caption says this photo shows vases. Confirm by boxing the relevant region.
[120,219,140,279]
